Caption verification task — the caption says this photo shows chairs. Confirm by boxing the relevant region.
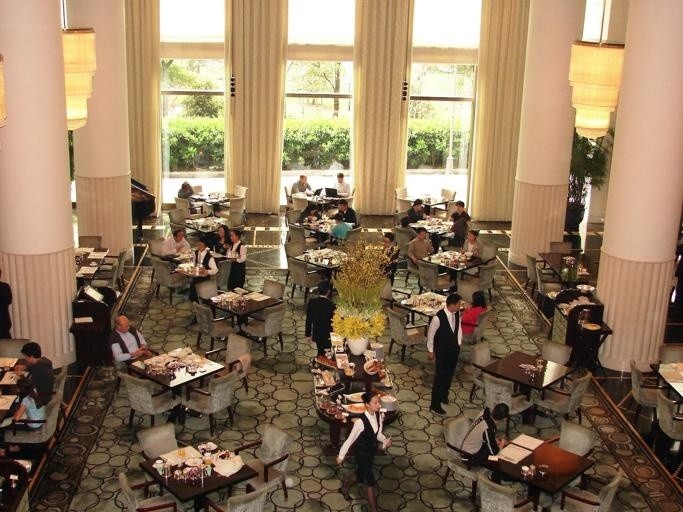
[443,415,623,510]
[117,423,292,510]
[0,362,71,510]
[67,234,127,294]
[470,340,593,437]
[119,333,252,435]
[629,343,681,459]
[525,240,593,311]
[148,186,500,362]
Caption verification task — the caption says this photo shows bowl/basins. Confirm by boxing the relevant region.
[363,358,380,375]
[576,284,595,293]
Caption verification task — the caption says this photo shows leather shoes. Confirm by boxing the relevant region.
[338,488,353,501]
[429,405,445,414]
[441,398,448,404]
[190,320,196,325]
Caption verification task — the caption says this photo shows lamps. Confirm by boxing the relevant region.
[565,38,625,141]
[62,27,97,131]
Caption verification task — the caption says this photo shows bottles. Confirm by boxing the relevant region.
[399,292,449,312]
[303,247,333,266]
[520,464,550,479]
[148,354,205,378]
[153,441,230,482]
[175,247,239,276]
[426,247,474,269]
[75,252,84,266]
[526,357,545,382]
[220,296,247,310]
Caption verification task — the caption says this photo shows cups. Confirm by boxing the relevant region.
[90,261,97,266]
[578,308,591,326]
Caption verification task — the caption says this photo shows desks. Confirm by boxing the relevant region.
[69,285,117,366]
[312,350,401,451]
[551,290,605,363]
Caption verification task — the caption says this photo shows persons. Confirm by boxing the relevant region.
[330,213,351,244]
[305,280,335,356]
[296,204,329,242]
[460,291,486,333]
[107,314,150,377]
[406,198,429,223]
[176,182,196,213]
[211,225,231,265]
[329,199,357,229]
[159,228,189,259]
[425,294,463,415]
[336,172,349,196]
[288,175,314,204]
[335,389,393,512]
[0,269,14,339]
[395,216,417,258]
[186,239,218,325]
[378,232,399,287]
[0,342,55,429]
[447,228,481,293]
[441,213,469,251]
[445,200,471,222]
[460,401,511,486]
[222,230,247,291]
[405,227,442,294]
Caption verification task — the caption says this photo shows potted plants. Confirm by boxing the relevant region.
[562,127,617,236]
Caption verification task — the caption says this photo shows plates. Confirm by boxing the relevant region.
[381,396,397,412]
[582,323,602,331]
[318,401,345,420]
[340,391,367,414]
